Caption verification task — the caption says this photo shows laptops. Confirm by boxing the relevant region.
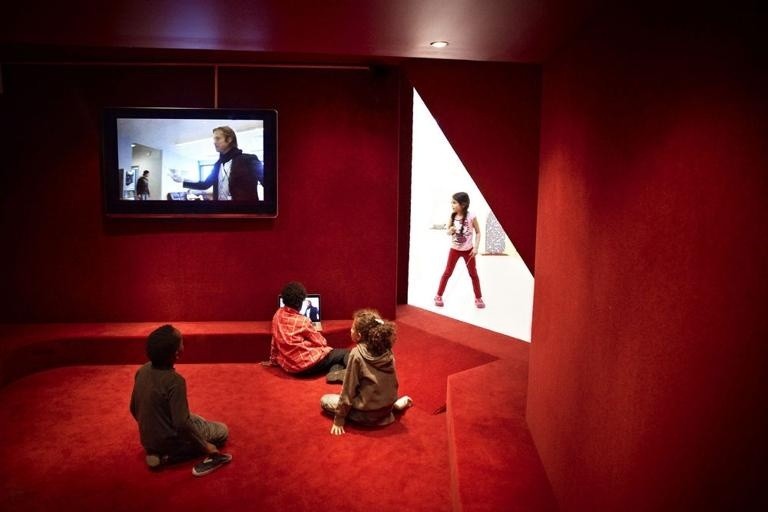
[278,294,322,331]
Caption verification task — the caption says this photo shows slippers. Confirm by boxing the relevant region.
[191,453,232,476]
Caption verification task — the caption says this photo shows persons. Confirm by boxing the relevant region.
[303,299,321,321]
[169,125,264,202]
[257,281,352,383]
[432,191,486,309]
[319,308,413,436]
[134,170,153,201]
[185,188,204,201]
[130,323,234,476]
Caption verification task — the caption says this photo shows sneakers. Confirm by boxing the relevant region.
[475,298,485,308]
[326,368,347,382]
[434,294,443,307]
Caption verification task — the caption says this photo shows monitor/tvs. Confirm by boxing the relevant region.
[100,106,283,220]
